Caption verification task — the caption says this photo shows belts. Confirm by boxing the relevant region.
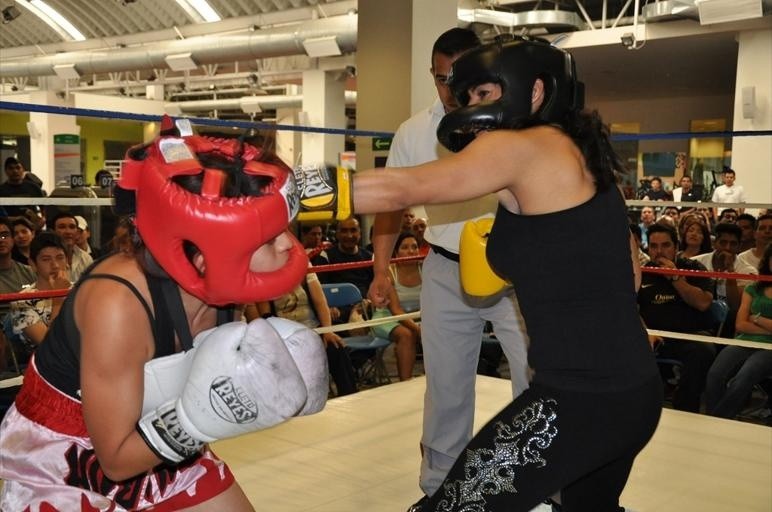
[429,243,459,263]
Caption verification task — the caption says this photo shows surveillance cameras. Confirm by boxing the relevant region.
[620,32,635,48]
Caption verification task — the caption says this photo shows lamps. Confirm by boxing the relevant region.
[618,30,639,51]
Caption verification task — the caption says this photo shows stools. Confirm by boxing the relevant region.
[477,322,503,374]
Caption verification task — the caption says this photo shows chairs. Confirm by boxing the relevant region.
[654,296,729,395]
[313,280,394,394]
[0,310,31,377]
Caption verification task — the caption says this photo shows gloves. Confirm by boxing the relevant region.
[458,215,512,297]
[140,315,333,420]
[133,316,312,469]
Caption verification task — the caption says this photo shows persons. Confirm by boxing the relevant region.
[365,25,561,511]
[275,35,662,511]
[1,132,337,511]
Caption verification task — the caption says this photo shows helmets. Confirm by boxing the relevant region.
[118,134,309,307]
[435,40,585,153]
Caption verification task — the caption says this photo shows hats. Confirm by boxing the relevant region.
[72,215,89,231]
